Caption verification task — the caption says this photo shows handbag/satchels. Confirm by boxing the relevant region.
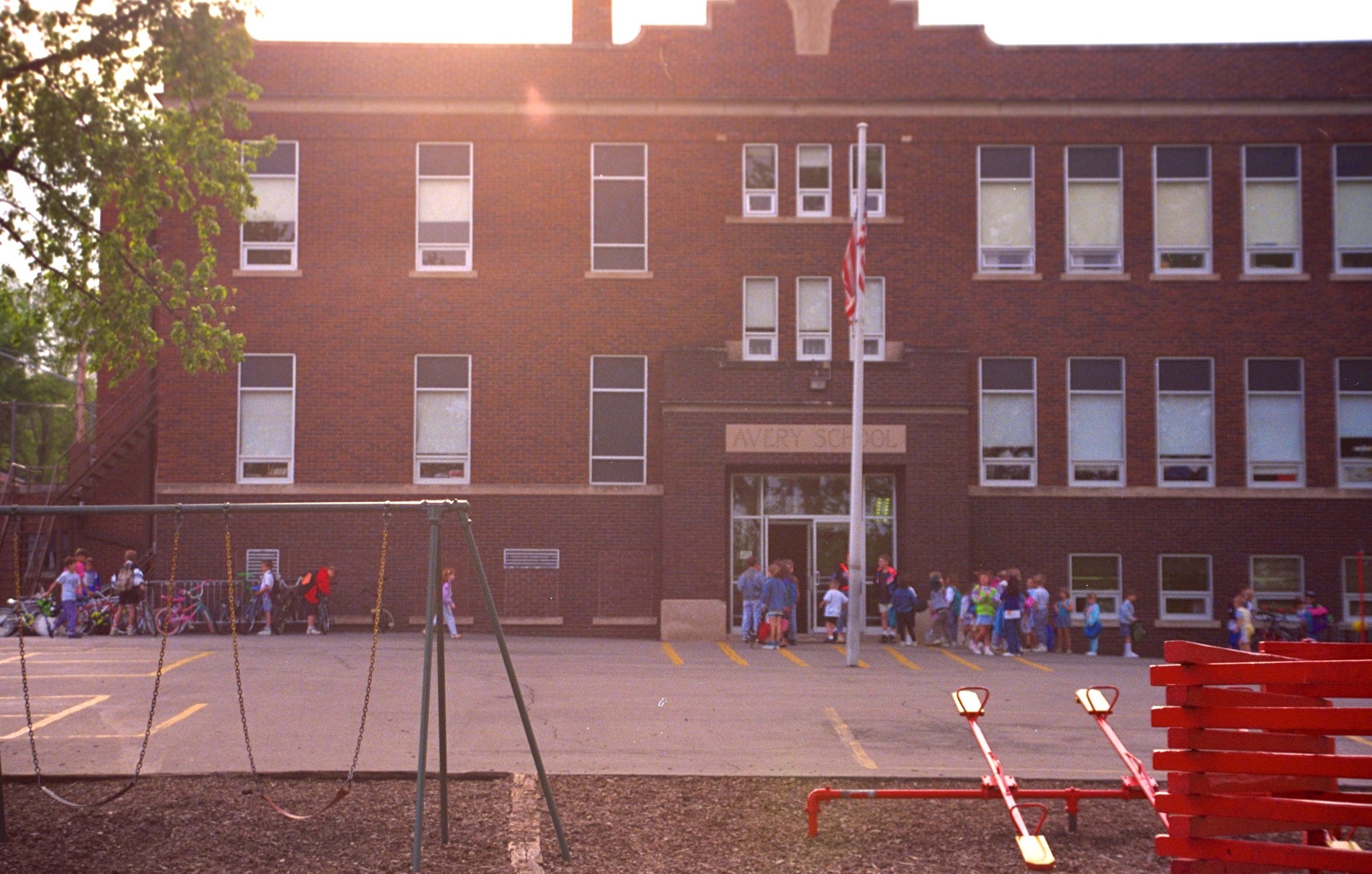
[1004,609,1021,620]
[782,617,790,632]
[758,613,770,641]
[888,606,898,628]
[1133,623,1146,644]
[1082,621,1103,638]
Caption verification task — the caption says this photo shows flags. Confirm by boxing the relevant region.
[839,191,870,321]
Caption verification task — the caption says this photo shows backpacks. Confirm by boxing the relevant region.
[950,585,962,616]
[267,573,280,603]
[115,567,136,592]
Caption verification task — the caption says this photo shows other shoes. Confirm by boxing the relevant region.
[900,640,919,647]
[1125,652,1139,657]
[48,625,84,638]
[743,635,797,649]
[257,628,271,636]
[306,629,323,635]
[933,639,1072,656]
[881,634,897,644]
[423,629,462,639]
[825,636,845,644]
[109,628,138,636]
[1086,652,1098,656]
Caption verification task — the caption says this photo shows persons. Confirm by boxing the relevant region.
[41,556,92,637]
[299,560,322,634]
[422,565,462,639]
[731,552,1349,669]
[253,559,275,635]
[61,547,94,632]
[108,549,145,635]
[82,556,102,601]
[315,565,337,633]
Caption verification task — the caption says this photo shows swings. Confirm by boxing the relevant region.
[223,500,392,819]
[11,503,185,808]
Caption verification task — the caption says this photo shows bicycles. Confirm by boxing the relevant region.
[213,572,303,635]
[1252,604,1309,651]
[154,579,215,635]
[360,578,394,633]
[315,581,330,634]
[0,583,188,638]
